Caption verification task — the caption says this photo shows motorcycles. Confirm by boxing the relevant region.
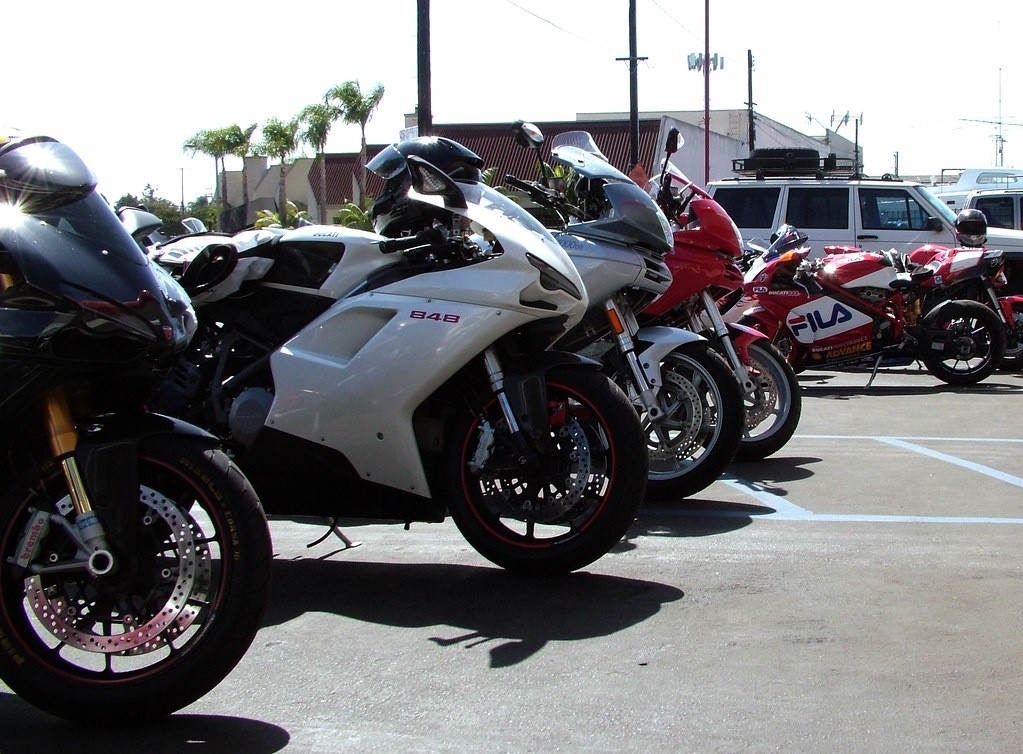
[124,121,1023,576]
[0,133,275,731]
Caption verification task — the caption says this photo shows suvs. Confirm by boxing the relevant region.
[701,158,1023,301]
[928,167,1023,255]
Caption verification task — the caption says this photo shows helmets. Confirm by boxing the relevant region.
[769,224,798,244]
[954,208,987,248]
[370,136,486,239]
